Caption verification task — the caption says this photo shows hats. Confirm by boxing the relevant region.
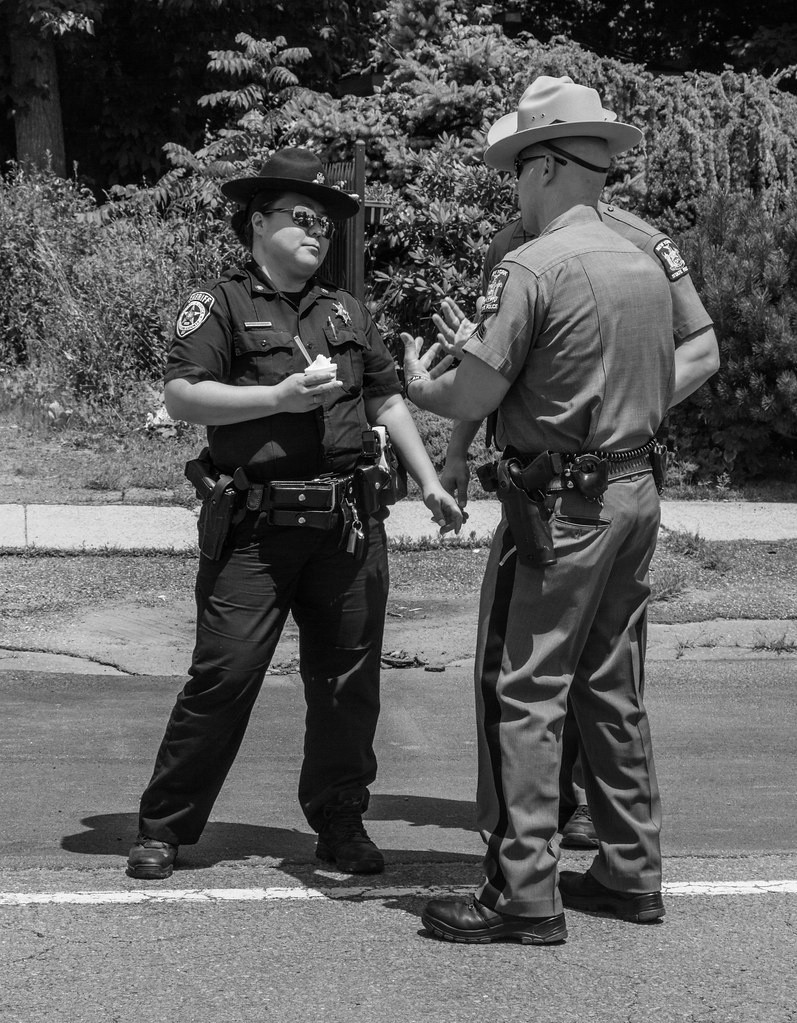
[483,75,644,172]
[221,148,359,221]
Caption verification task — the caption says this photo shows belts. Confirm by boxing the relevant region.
[229,472,362,514]
[543,450,653,493]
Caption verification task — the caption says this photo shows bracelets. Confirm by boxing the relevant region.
[405,376,430,403]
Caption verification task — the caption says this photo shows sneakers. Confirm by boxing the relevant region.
[562,803,600,848]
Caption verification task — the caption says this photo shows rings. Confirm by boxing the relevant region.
[314,395,317,404]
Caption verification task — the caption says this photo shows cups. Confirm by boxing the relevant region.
[305,364,337,384]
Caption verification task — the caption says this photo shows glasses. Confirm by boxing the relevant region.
[514,156,567,180]
[263,204,335,239]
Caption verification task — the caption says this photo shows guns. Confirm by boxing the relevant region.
[501,449,562,503]
[184,458,235,505]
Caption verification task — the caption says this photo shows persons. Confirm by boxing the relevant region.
[400,76,720,943]
[128,148,463,877]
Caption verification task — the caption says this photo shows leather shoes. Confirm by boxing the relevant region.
[557,871,665,923]
[127,832,179,878]
[421,892,568,945]
[316,813,385,874]
